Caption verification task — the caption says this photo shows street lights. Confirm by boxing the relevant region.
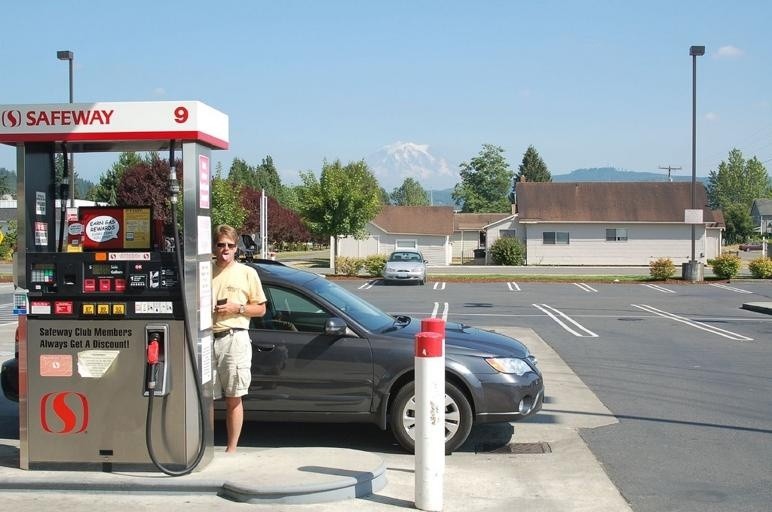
[56,50,75,208]
[681,45,705,283]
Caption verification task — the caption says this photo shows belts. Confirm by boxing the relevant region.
[213,328,245,338]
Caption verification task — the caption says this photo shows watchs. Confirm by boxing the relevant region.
[237,303,245,315]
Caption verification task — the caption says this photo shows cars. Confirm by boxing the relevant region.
[739,242,768,252]
[2,258,545,455]
[381,249,429,285]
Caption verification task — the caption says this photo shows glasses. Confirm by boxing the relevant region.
[217,242,236,248]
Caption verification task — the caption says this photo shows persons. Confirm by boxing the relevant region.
[212,222,268,452]
